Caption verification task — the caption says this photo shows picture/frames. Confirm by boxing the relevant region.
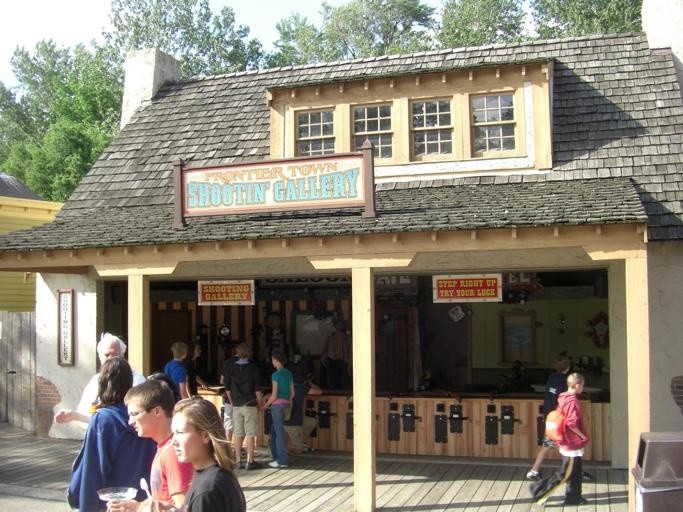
[497,306,537,367]
[56,289,76,369]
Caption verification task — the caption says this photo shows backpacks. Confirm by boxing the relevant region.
[545,411,565,441]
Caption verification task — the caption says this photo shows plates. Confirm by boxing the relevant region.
[96,486,138,502]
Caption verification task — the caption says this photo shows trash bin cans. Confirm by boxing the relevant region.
[630,432,683,512]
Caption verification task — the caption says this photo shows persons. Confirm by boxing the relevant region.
[525,350,597,483]
[556,371,590,505]
[56,319,351,512]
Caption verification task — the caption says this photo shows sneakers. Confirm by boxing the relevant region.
[526,469,592,505]
[232,442,305,469]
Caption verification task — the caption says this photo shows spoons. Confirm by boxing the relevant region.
[139,477,150,500]
